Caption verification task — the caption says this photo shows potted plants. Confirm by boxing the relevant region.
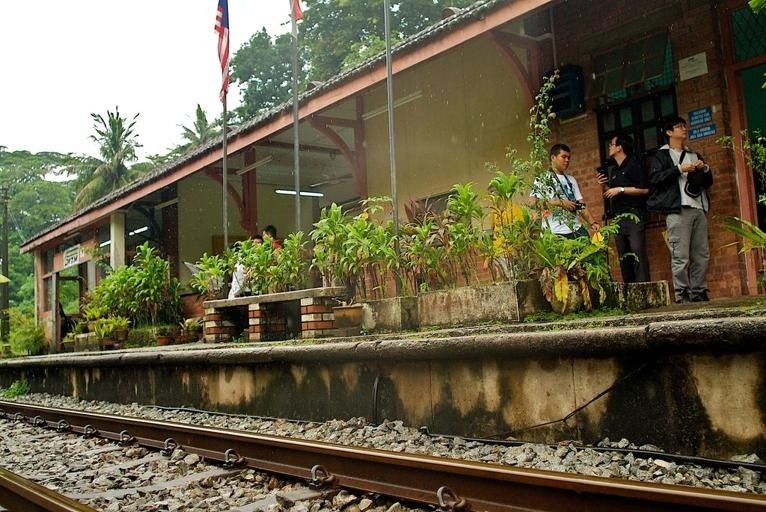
[521,229,604,314]
[155,316,203,346]
[60,304,130,351]
[309,204,363,336]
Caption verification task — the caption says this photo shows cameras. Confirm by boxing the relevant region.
[570,200,585,212]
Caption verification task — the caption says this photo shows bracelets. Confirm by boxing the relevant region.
[590,220,600,227]
[621,186,625,194]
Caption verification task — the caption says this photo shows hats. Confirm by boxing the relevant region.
[684,166,705,198]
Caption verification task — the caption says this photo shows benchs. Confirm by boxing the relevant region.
[200,284,346,342]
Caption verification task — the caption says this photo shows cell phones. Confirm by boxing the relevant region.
[596,166,608,180]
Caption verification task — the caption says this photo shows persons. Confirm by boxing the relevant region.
[250,234,263,248]
[592,129,654,282]
[528,140,602,282]
[261,224,282,251]
[645,111,715,303]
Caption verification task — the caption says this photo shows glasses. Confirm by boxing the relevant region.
[673,124,687,128]
[608,142,617,146]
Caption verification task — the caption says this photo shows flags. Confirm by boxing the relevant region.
[214,1,233,102]
